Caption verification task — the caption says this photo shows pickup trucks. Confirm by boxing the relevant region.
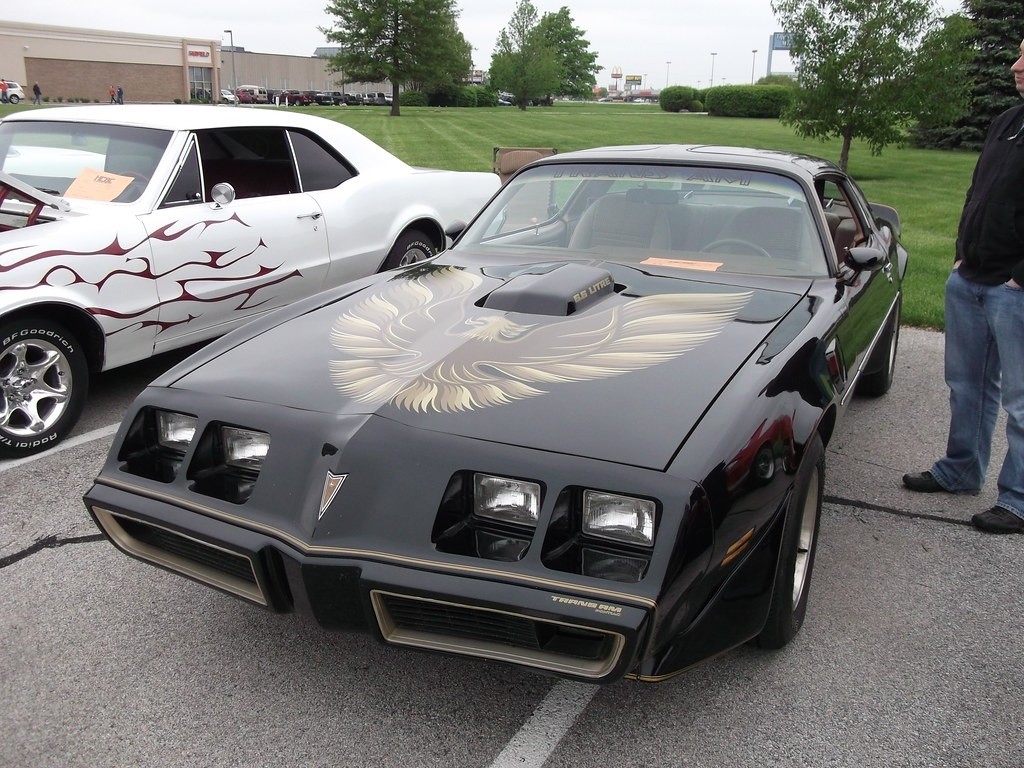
[278,89,315,106]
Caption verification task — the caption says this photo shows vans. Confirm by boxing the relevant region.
[235,85,266,103]
[362,92,386,105]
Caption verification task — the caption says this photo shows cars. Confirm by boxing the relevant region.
[267,87,321,103]
[315,91,345,105]
[231,89,252,103]
[598,97,613,102]
[344,93,363,105]
[383,95,393,105]
[221,89,239,104]
[0,81,26,104]
[499,98,512,106]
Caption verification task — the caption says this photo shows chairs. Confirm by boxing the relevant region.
[493,147,558,184]
[712,208,811,262]
[572,192,669,252]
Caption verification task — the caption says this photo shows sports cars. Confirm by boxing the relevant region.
[0,105,506,458]
[79,143,910,686]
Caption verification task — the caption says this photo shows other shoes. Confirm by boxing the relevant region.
[902,471,944,492]
[972,505,1024,533]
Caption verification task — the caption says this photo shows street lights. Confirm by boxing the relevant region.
[224,29,238,108]
[644,73,648,91]
[710,53,717,88]
[666,61,671,88]
[721,77,727,86]
[697,80,700,90]
[751,49,758,85]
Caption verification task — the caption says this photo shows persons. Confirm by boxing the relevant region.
[117,84,124,104]
[110,85,117,103]
[33,82,42,105]
[903,39,1024,533]
[0,79,9,102]
